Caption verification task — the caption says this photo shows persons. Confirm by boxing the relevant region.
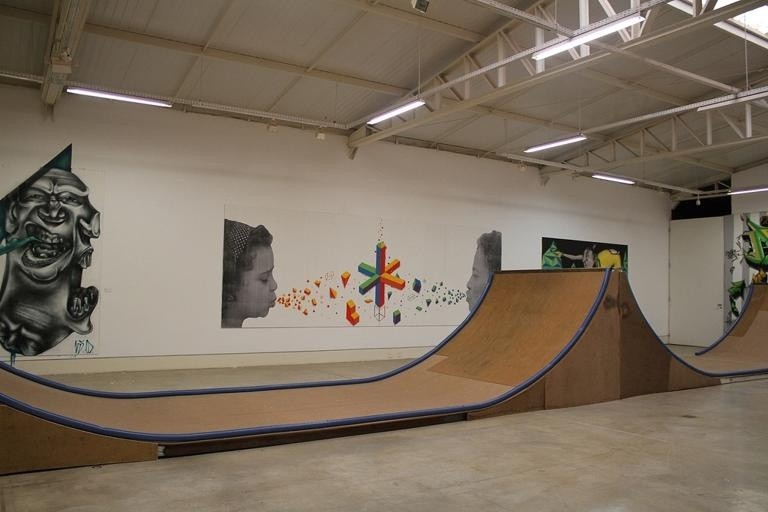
[465,229,502,312]
[220,218,279,329]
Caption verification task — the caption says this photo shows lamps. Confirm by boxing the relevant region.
[410,0,429,15]
[366,98,425,125]
[525,90,768,195]
[66,88,173,108]
[532,6,646,62]
